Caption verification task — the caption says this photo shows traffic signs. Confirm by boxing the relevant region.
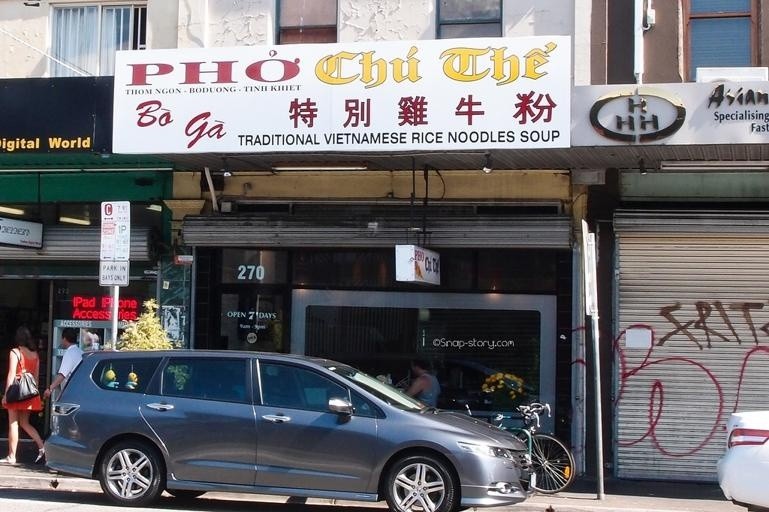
[100,201,130,261]
[99,261,130,287]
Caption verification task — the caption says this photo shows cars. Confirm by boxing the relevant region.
[715,410,769,512]
[44,349,537,512]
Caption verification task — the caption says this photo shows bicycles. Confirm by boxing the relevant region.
[449,400,576,494]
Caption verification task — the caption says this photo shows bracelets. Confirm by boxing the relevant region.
[48,386,53,393]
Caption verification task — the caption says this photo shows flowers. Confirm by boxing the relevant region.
[482,373,524,399]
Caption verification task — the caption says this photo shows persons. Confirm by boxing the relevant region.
[405,355,442,409]
[42,326,87,473]
[0,325,49,467]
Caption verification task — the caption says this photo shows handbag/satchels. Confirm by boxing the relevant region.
[6,349,39,403]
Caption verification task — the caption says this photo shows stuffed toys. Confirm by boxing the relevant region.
[103,368,120,388]
[124,371,139,389]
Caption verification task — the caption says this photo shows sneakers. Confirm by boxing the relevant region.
[35,447,44,463]
[0,456,16,465]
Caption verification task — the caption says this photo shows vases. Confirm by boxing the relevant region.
[492,391,512,412]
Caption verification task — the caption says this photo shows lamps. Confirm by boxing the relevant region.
[89,113,109,158]
[0,204,24,215]
[58,203,91,226]
[639,158,647,175]
[482,154,494,174]
[660,161,769,170]
